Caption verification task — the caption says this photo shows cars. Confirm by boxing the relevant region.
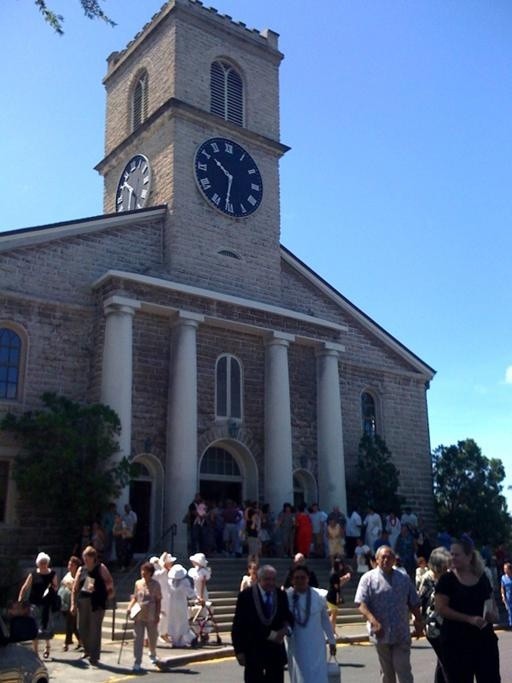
[0,611,50,682]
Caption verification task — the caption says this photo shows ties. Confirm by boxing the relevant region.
[265,591,274,620]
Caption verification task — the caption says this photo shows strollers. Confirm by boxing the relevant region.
[188,597,223,645]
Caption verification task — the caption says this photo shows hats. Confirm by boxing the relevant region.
[189,553,207,568]
[159,553,188,580]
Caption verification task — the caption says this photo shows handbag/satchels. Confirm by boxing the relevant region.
[326,661,343,682]
[424,592,449,638]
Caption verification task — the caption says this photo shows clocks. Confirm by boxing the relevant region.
[114,151,153,211]
[195,138,265,216]
